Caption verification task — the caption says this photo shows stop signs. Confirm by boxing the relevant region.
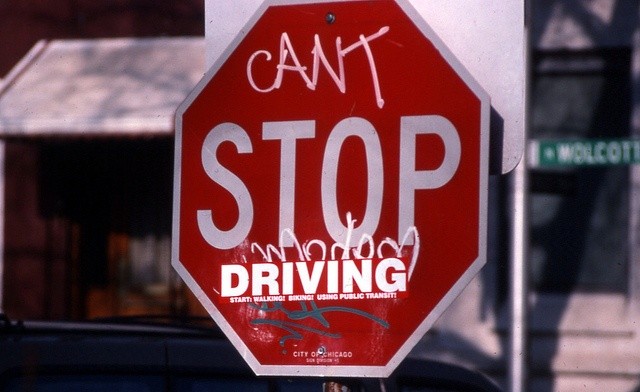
[171,1,490,378]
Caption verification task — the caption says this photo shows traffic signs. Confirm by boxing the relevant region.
[528,139,640,168]
